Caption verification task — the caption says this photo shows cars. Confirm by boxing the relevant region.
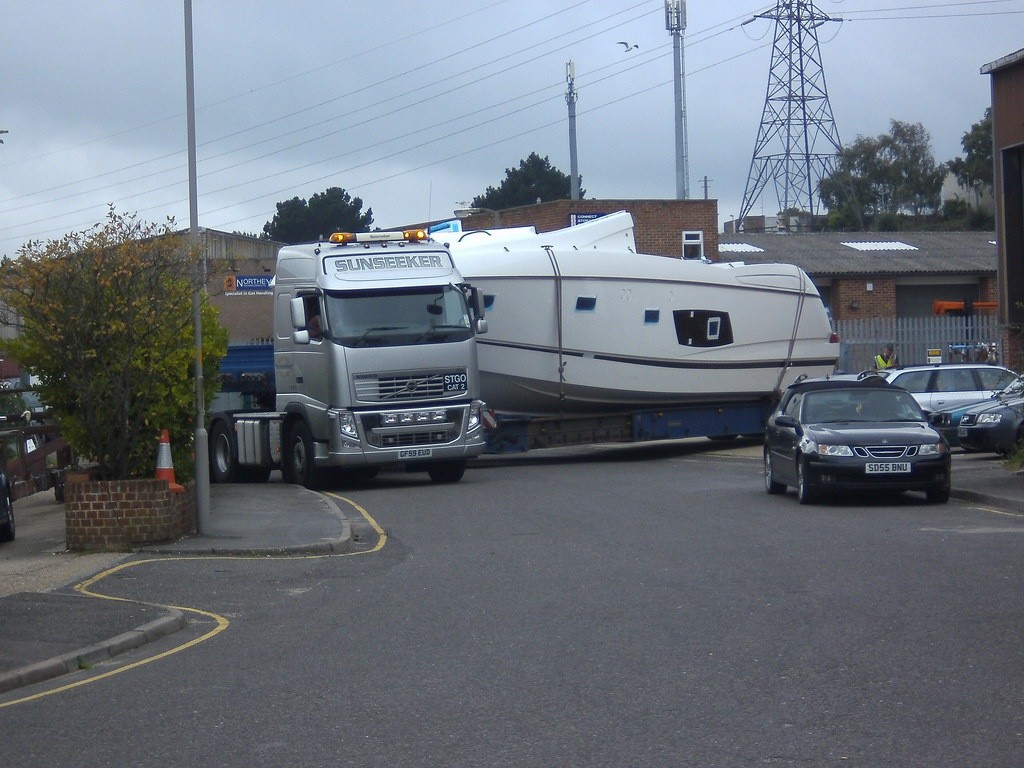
[956,375,1024,457]
[873,364,1022,443]
[764,370,951,506]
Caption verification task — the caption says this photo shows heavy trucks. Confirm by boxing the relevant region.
[197,229,497,487]
[0,301,90,544]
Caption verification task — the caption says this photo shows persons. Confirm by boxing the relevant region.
[21,407,35,426]
[911,374,925,392]
[872,343,900,370]
[310,314,322,338]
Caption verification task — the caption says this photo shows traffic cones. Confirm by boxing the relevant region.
[153,428,185,492]
